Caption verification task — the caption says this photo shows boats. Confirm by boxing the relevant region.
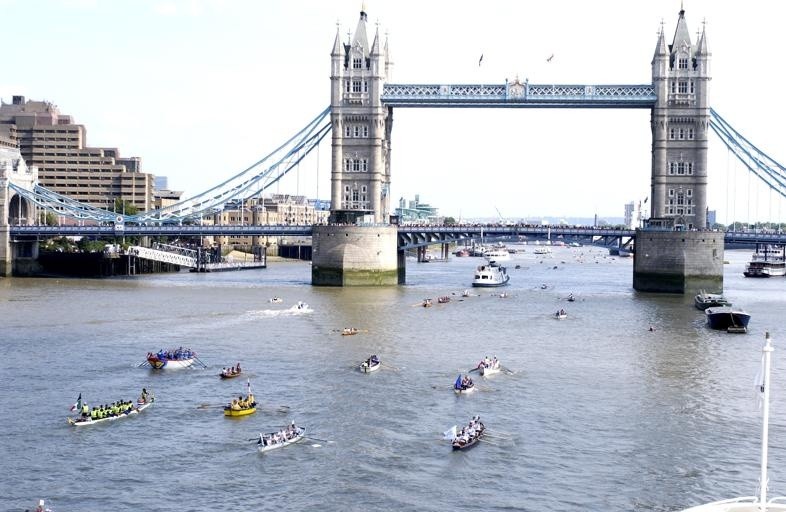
[454,374,475,393]
[541,283,576,319]
[424,289,470,306]
[695,292,731,309]
[148,351,197,368]
[500,292,507,297]
[469,242,509,287]
[219,363,242,378]
[341,328,368,335]
[257,421,306,452]
[534,246,552,255]
[271,296,307,311]
[452,416,485,448]
[744,241,785,277]
[480,365,502,376]
[705,304,750,333]
[360,354,380,372]
[223,378,258,416]
[67,393,154,425]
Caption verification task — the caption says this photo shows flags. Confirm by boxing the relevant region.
[70,393,81,412]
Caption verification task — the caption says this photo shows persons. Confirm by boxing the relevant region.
[222,362,241,375]
[556,310,560,316]
[344,328,347,332]
[79,388,149,420]
[231,393,256,410]
[362,362,369,367]
[366,358,372,367]
[39,505,43,512]
[561,307,565,315]
[158,347,193,360]
[271,424,300,444]
[453,354,498,444]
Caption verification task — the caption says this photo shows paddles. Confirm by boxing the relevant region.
[299,436,335,444]
[256,404,290,413]
[196,404,229,409]
[288,442,323,448]
